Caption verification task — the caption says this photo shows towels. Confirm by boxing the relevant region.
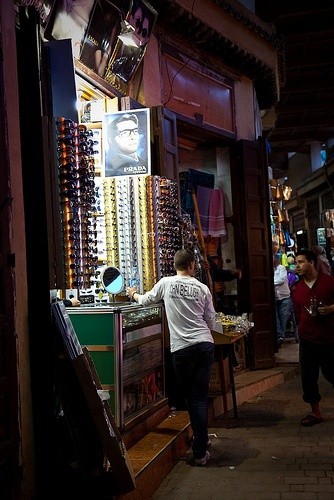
[192,185,212,236]
[208,188,226,237]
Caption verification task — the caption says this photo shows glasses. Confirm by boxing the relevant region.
[54,118,182,302]
[116,128,140,137]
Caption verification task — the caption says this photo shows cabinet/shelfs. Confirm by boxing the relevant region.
[207,329,249,429]
[66,301,168,431]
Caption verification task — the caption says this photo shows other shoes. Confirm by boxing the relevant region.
[206,439,213,448]
[277,338,285,344]
[195,451,210,466]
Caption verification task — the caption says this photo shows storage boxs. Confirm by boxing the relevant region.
[210,357,231,392]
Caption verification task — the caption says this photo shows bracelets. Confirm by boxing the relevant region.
[131,292,137,299]
[104,50,110,59]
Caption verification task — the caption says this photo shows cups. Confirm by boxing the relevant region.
[310,296,322,317]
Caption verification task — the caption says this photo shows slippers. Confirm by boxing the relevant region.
[301,415,323,426]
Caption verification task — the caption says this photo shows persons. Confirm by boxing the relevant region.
[63,298,80,307]
[273,245,334,344]
[105,114,147,177]
[291,250,334,427]
[127,249,216,465]
[93,15,117,77]
